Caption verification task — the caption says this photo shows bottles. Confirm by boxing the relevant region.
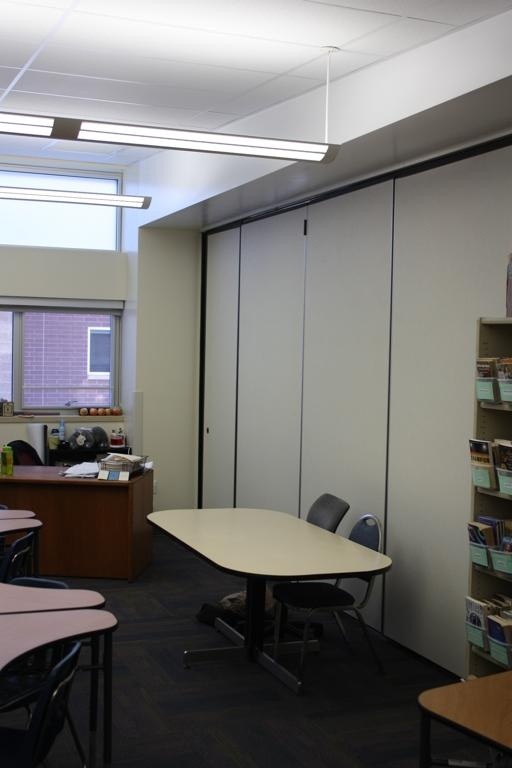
[59,419,65,444]
[109,428,128,448]
[52,429,59,435]
[0,443,14,476]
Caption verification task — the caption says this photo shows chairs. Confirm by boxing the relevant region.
[238,493,390,683]
[4,439,46,465]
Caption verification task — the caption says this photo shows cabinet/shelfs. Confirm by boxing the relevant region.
[467,311,511,682]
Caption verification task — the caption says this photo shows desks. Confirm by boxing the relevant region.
[419,669,512,768]
[146,507,393,689]
[0,463,153,583]
[0,505,118,768]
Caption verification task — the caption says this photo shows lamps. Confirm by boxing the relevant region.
[0,183,152,209]
[0,45,341,164]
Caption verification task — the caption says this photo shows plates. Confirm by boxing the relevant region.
[19,415,34,418]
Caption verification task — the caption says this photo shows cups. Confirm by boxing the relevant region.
[48,436,58,450]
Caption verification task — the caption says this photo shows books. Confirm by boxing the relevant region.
[464,346,512,647]
